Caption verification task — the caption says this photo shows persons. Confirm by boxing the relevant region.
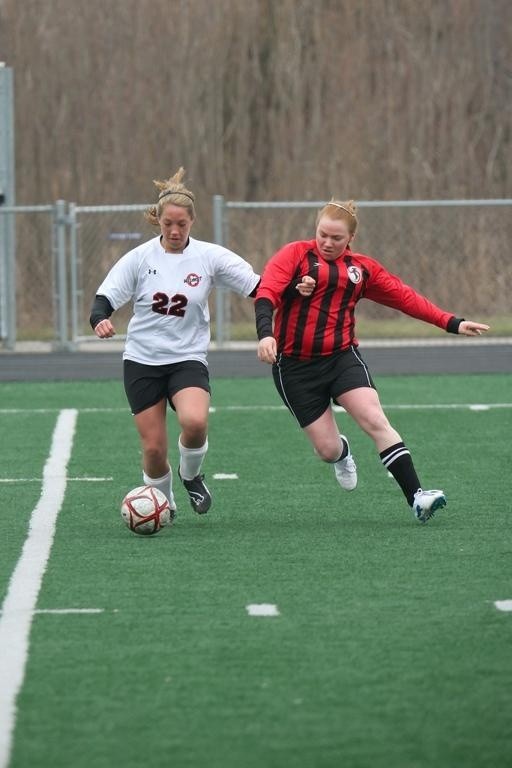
[88,167,318,518]
[251,197,492,526]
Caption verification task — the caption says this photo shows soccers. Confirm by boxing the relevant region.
[121,486,172,535]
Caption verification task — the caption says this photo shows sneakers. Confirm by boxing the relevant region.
[412,487,446,521]
[334,435,357,490]
[178,463,212,514]
